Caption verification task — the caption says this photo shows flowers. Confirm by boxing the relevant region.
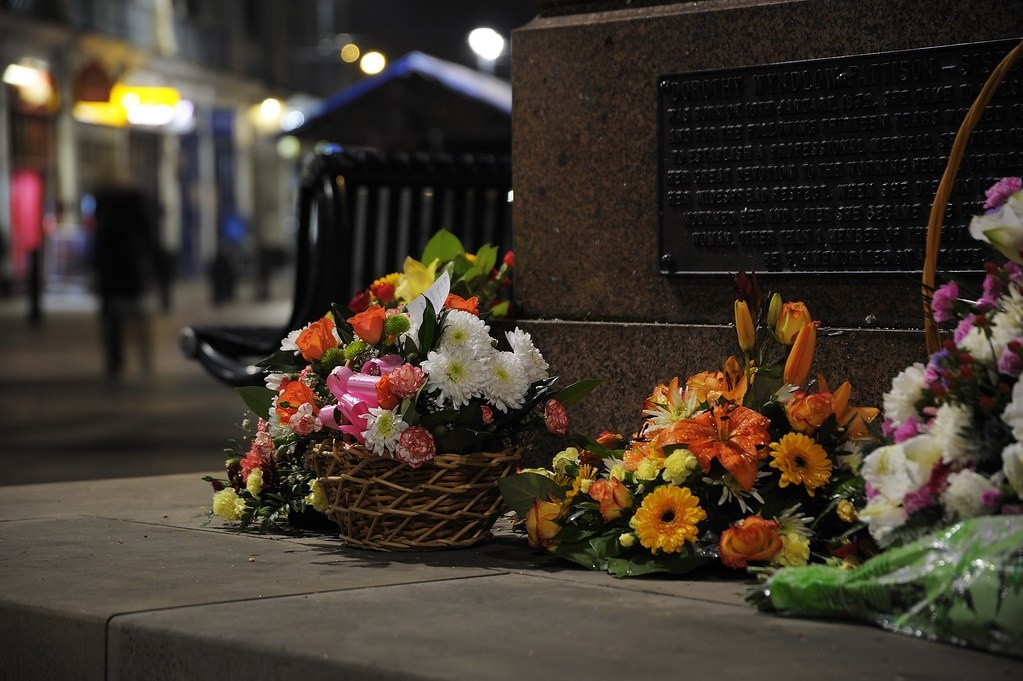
[503,289,883,579]
[207,256,572,533]
[859,176,1023,551]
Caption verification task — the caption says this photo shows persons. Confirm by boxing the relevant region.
[76,153,172,381]
